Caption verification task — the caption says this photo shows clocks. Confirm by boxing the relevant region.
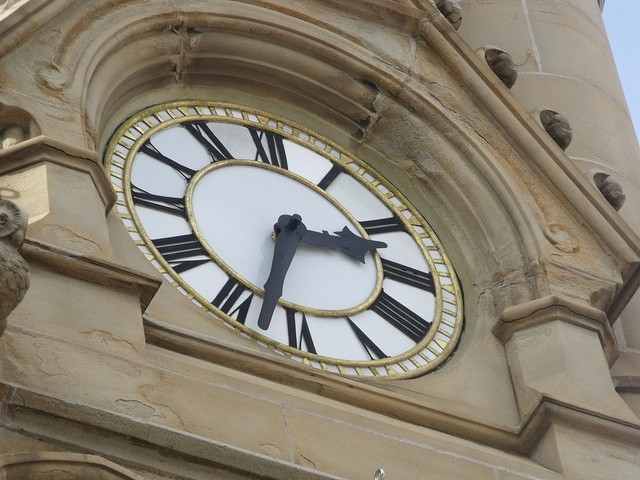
[103,100,464,379]
